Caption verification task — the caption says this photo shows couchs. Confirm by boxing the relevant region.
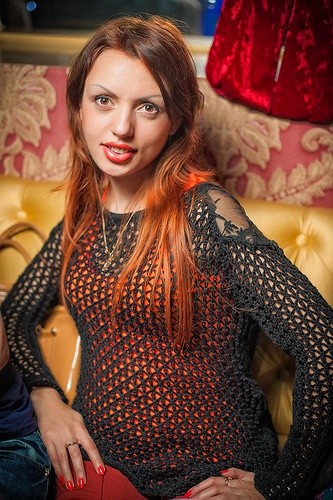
[0,174,331,454]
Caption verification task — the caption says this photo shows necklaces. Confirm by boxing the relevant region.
[100,189,147,272]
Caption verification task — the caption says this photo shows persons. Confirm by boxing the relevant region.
[0,292,52,500]
[2,18,331,499]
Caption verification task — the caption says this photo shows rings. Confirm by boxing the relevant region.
[66,440,77,451]
[224,474,233,488]
